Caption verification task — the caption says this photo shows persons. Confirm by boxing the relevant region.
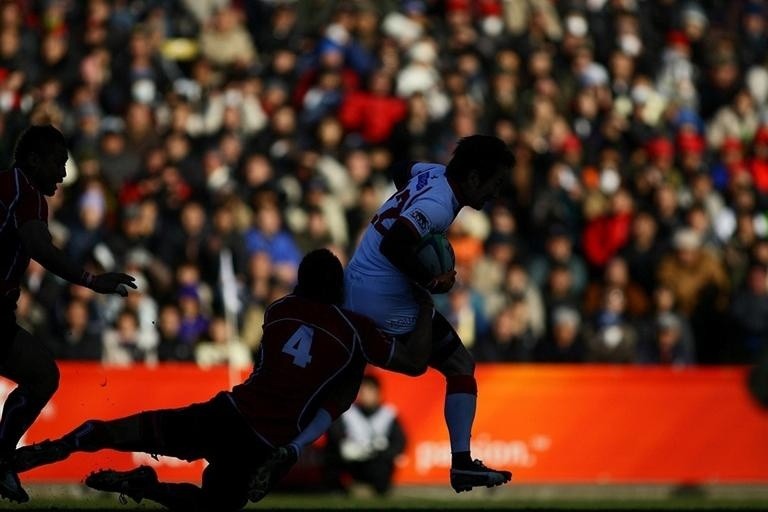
[1,123,137,501]
[347,135,514,493]
[16,247,365,512]
[1,1,768,364]
[343,373,407,498]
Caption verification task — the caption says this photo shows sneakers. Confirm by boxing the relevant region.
[16,442,68,473]
[85,464,158,504]
[449,459,513,493]
[248,441,298,501]
[1,463,29,505]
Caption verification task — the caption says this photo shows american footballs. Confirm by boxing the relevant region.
[414,235,455,279]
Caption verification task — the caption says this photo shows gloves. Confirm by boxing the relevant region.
[94,271,137,299]
[426,269,457,296]
